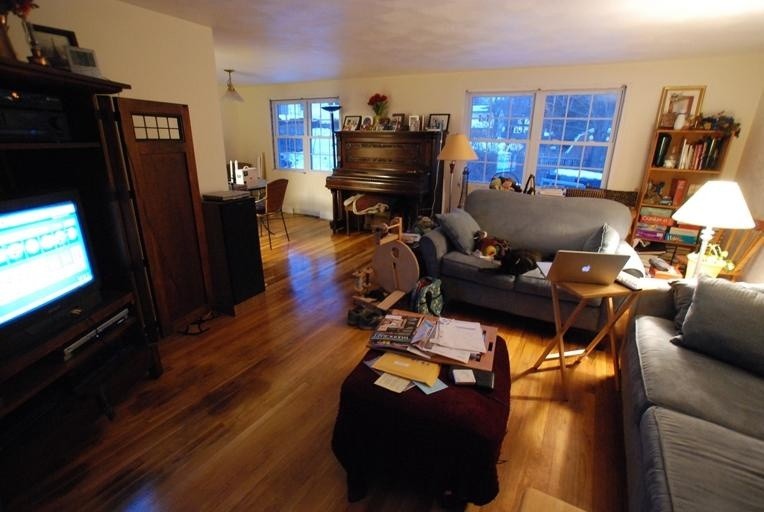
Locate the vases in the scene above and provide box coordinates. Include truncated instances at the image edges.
[685,253,726,281]
[674,113,685,130]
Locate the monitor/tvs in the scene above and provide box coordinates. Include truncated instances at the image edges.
[0,188,103,362]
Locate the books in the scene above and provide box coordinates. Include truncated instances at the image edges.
[635,127,726,278]
[358,310,498,396]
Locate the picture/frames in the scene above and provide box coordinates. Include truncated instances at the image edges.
[341,112,450,132]
[656,85,706,130]
[26,23,80,56]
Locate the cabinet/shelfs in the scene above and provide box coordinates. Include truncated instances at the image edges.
[630,130,734,279]
[0,56,161,444]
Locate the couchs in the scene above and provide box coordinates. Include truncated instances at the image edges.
[619,283,764,512]
[419,190,645,351]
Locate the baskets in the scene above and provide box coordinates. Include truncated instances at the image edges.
[565,188,606,198]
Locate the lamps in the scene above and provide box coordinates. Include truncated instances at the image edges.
[671,180,757,279]
[219,70,245,103]
[320,106,343,227]
[434,133,479,214]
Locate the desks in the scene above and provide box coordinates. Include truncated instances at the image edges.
[229,180,270,237]
[533,279,671,403]
[331,317,511,511]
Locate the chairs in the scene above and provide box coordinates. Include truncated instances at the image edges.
[675,219,764,283]
[226,162,251,184]
[256,178,289,252]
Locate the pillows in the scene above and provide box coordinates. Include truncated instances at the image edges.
[583,222,621,254]
[670,274,764,381]
[434,208,484,257]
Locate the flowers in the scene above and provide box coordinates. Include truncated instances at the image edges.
[0,0,39,21]
[367,93,390,116]
[693,243,735,272]
[490,177,523,192]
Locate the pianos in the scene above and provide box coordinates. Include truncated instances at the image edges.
[324,129,450,236]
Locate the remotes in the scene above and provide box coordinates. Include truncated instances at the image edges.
[616,271,644,291]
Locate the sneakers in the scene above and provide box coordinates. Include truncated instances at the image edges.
[359,309,383,330]
[348,303,371,325]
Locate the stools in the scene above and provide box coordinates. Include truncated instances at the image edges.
[648,264,684,279]
[344,204,391,237]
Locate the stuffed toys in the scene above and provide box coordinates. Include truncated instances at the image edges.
[472,230,489,252]
[479,239,513,260]
[490,170,536,196]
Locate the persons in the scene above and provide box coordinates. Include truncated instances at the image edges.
[362,117,372,128]
[391,116,401,126]
[382,118,395,130]
[348,119,359,128]
[346,118,351,128]
[410,118,418,130]
[432,116,444,129]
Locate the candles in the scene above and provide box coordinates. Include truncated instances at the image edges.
[229,159,239,182]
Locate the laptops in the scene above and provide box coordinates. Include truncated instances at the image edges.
[536,250,631,285]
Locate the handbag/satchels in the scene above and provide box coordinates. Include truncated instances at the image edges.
[410,276,444,316]
[493,248,538,275]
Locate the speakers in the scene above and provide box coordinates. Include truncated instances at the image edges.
[200,196,266,305]
